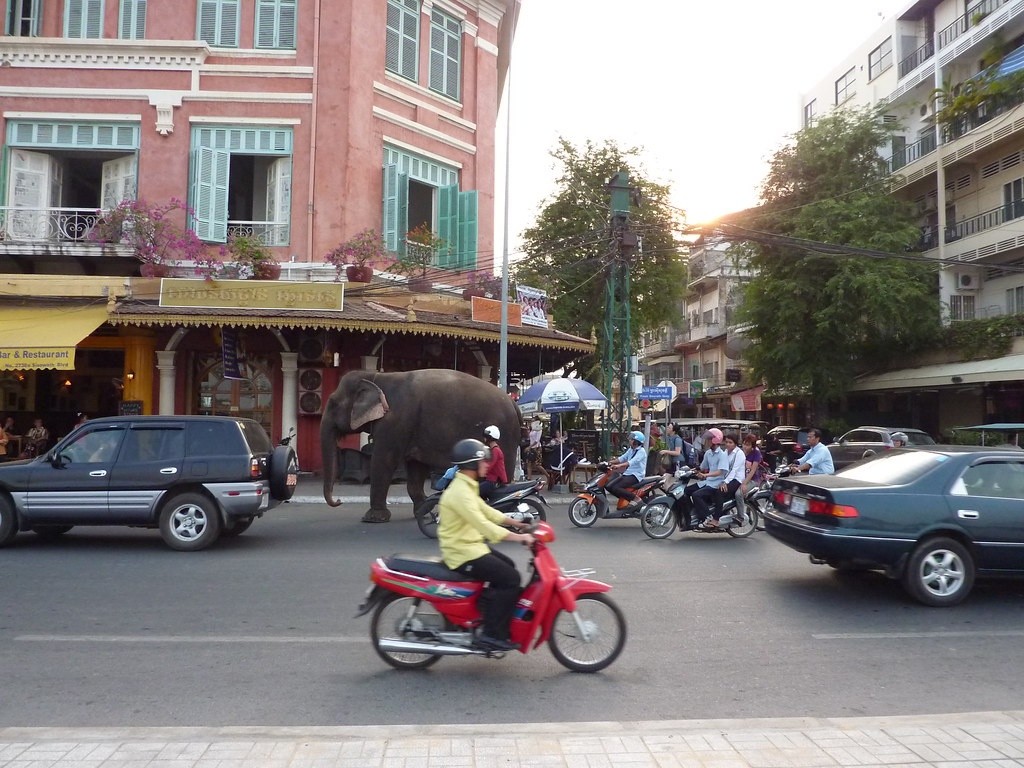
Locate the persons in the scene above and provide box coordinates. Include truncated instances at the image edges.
[478,425,508,501]
[437,439,539,652]
[0,416,48,462]
[605,431,648,511]
[889,432,909,447]
[89,435,118,466]
[520,297,546,319]
[521,415,577,491]
[788,428,835,477]
[73,414,92,448]
[658,422,788,530]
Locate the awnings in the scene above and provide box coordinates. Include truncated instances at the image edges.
[0,300,111,371]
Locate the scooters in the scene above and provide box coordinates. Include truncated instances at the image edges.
[275,427,296,447]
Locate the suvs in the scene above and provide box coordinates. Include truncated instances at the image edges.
[0,416,298,553]
[825,425,937,472]
[756,425,833,477]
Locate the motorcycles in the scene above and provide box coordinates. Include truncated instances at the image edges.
[746,456,800,530]
[351,501,628,674]
[416,466,551,541]
[639,464,759,540]
[567,455,667,527]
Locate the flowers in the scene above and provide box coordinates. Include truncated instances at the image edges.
[320,221,447,291]
[464,269,515,301]
[75,186,279,282]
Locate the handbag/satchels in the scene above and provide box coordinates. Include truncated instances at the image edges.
[662,453,672,469]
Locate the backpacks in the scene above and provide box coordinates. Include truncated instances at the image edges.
[674,436,700,469]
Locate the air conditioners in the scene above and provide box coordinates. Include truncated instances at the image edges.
[298,368,323,415]
[957,273,983,292]
[299,334,325,362]
[907,190,955,217]
[919,101,934,122]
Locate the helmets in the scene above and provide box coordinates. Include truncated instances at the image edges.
[451,439,490,470]
[890,431,908,442]
[484,425,501,439]
[629,431,645,444]
[703,428,723,445]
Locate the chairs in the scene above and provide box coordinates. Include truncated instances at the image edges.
[544,449,576,491]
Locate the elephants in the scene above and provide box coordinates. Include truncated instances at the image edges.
[319,367,530,524]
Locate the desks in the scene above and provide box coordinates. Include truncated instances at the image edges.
[7,435,26,459]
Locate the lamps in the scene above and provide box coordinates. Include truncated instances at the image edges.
[127,368,135,383]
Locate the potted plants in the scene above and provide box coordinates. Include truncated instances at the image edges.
[927,63,1024,138]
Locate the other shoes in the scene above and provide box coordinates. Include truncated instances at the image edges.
[698,514,713,528]
[706,519,721,527]
[628,499,643,508]
[479,633,520,650]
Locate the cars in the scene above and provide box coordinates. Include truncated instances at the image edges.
[763,446,1023,610]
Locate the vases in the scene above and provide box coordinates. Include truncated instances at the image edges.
[463,289,485,301]
[141,263,169,279]
[346,265,373,282]
[253,263,282,280]
[408,278,433,294]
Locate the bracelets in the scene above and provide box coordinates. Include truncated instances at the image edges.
[99,446,103,451]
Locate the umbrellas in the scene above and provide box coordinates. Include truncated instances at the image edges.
[517,376,610,485]
